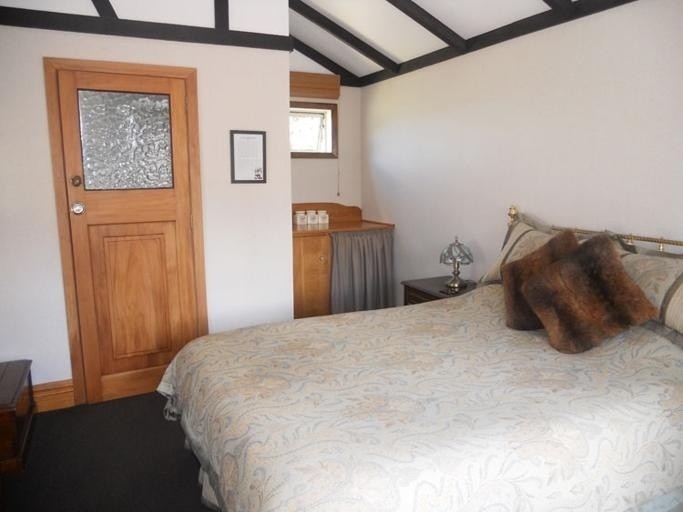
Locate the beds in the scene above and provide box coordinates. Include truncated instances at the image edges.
[157,206,683,511]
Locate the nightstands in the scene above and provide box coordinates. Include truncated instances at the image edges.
[401,276,477,307]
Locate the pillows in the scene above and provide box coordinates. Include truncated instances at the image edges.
[477,219,683,341]
[522,236,657,352]
[504,236,578,329]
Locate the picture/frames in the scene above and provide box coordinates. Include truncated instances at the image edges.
[230,131,265,183]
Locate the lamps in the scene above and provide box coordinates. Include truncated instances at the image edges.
[439,236,472,287]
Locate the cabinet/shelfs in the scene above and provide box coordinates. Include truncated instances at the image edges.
[293,202,394,317]
[0,360,37,477]
